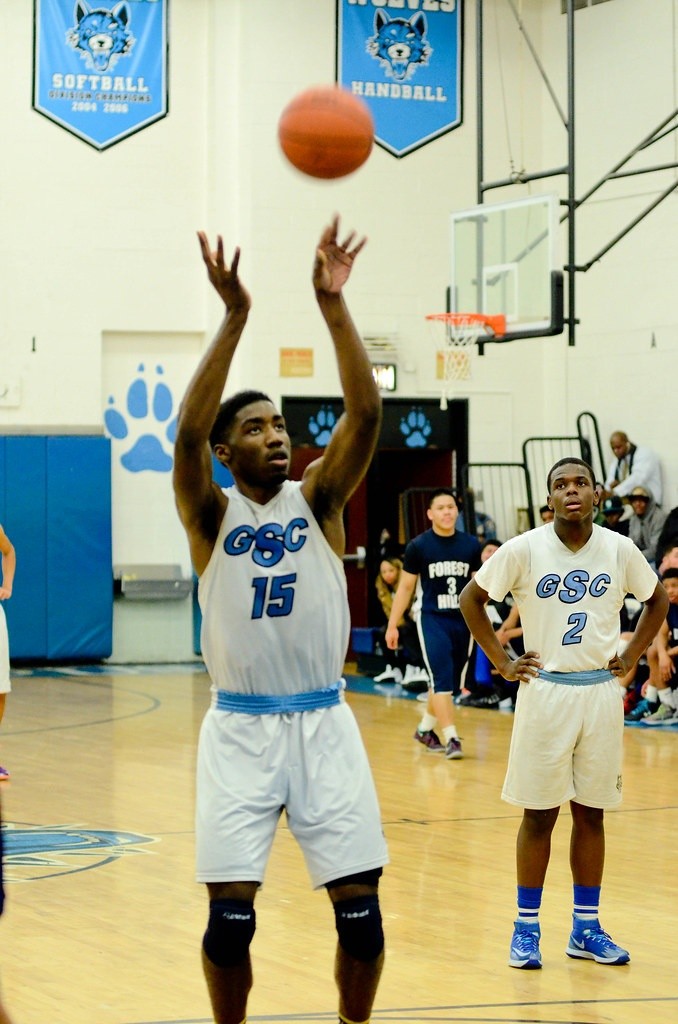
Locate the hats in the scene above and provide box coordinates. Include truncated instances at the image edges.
[600,496,625,515]
[628,487,651,503]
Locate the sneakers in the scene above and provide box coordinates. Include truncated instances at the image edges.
[0,767,10,780]
[400,664,430,702]
[624,695,661,724]
[509,917,544,970]
[460,688,512,710]
[412,728,446,753]
[373,664,403,683]
[640,702,678,726]
[567,914,631,964]
[624,681,643,716]
[445,736,464,759]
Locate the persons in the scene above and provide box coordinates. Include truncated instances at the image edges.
[374,431,678,727]
[458,458,669,971]
[173,214,384,1023]
[384,487,483,760]
[0,525,15,780]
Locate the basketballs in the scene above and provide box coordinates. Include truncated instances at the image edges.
[278,87,375,178]
